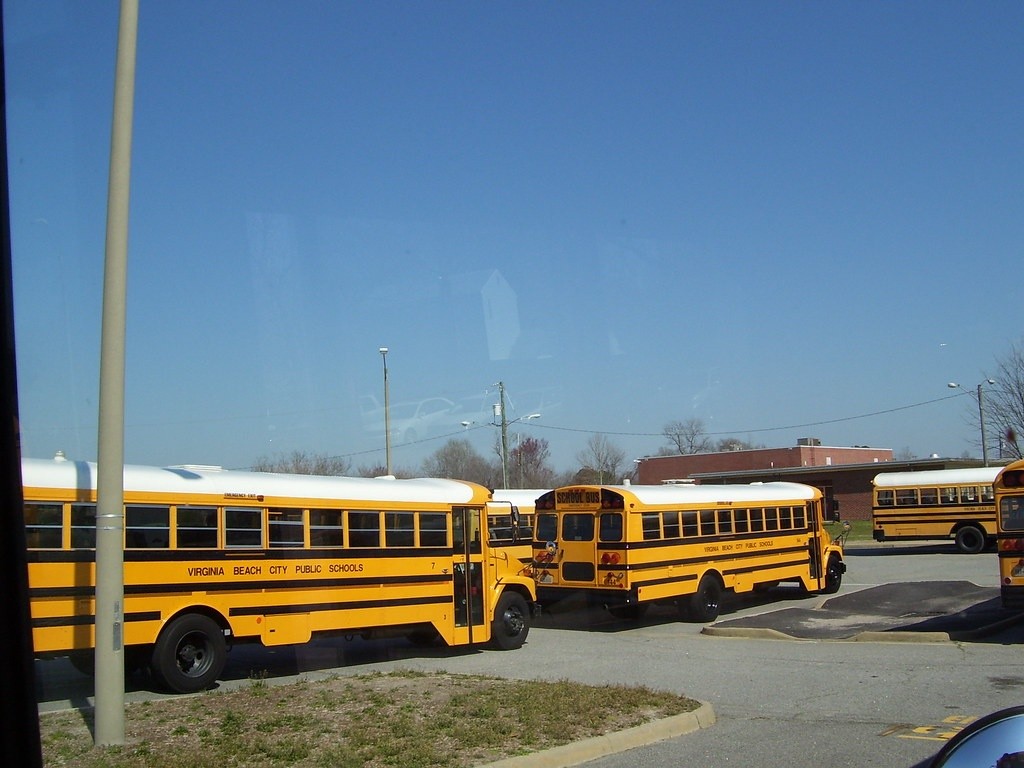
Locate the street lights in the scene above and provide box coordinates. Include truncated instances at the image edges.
[379,346,392,478]
[947,378,996,466]
[462,414,543,488]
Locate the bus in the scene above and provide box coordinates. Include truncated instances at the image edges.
[871,466,1016,554]
[470,484,584,596]
[21,456,540,695]
[531,477,852,626]
[991,457,1024,611]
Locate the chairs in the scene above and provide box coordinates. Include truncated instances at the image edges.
[880,495,988,504]
